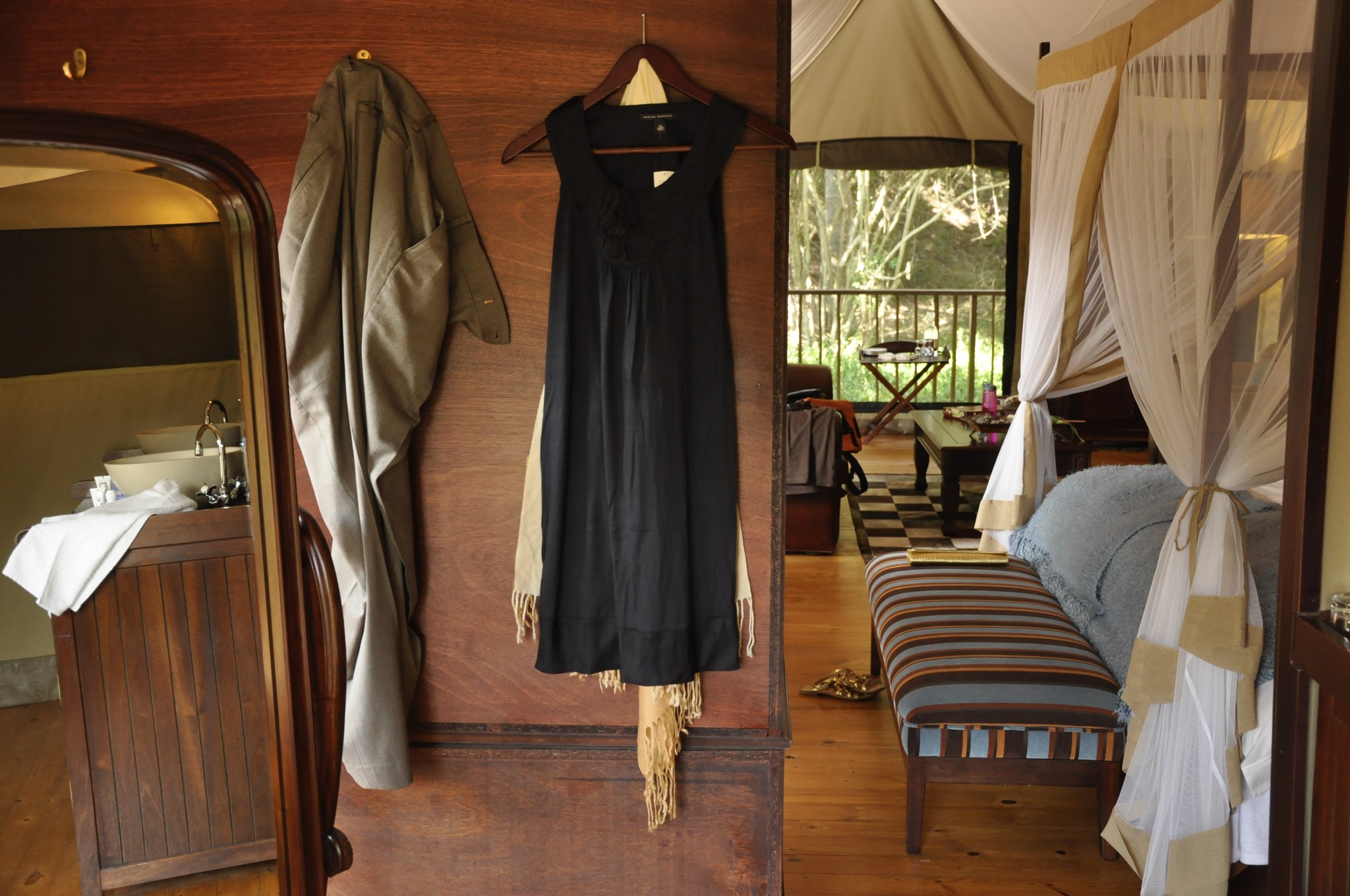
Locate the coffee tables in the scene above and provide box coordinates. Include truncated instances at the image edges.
[907,404,1099,538]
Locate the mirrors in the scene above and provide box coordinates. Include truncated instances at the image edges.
[0,99,358,896]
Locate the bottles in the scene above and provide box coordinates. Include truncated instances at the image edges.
[982,381,997,417]
[923,321,939,356]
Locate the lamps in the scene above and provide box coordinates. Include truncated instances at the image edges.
[923,324,939,358]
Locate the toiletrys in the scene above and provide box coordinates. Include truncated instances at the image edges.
[89,475,125,507]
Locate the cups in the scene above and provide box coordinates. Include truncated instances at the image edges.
[1329,592,1350,637]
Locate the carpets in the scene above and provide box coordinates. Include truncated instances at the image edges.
[844,472,991,566]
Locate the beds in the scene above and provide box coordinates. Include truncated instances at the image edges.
[1003,0,1350,896]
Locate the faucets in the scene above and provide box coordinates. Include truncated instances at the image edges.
[204,397,230,426]
[193,423,231,506]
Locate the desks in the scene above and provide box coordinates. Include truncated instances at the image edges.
[856,350,950,447]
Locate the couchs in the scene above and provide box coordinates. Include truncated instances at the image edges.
[781,360,844,559]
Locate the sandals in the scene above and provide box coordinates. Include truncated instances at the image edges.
[801,667,885,700]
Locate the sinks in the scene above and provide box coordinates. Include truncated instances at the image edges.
[102,444,246,498]
[133,421,241,455]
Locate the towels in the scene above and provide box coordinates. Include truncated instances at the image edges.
[1,477,199,617]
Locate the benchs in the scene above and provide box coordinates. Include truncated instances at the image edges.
[861,547,1128,863]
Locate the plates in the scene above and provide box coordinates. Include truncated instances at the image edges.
[863,348,887,356]
[960,414,1015,433]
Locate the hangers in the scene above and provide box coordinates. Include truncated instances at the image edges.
[493,13,798,167]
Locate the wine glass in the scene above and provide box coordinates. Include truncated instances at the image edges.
[914,340,934,356]
